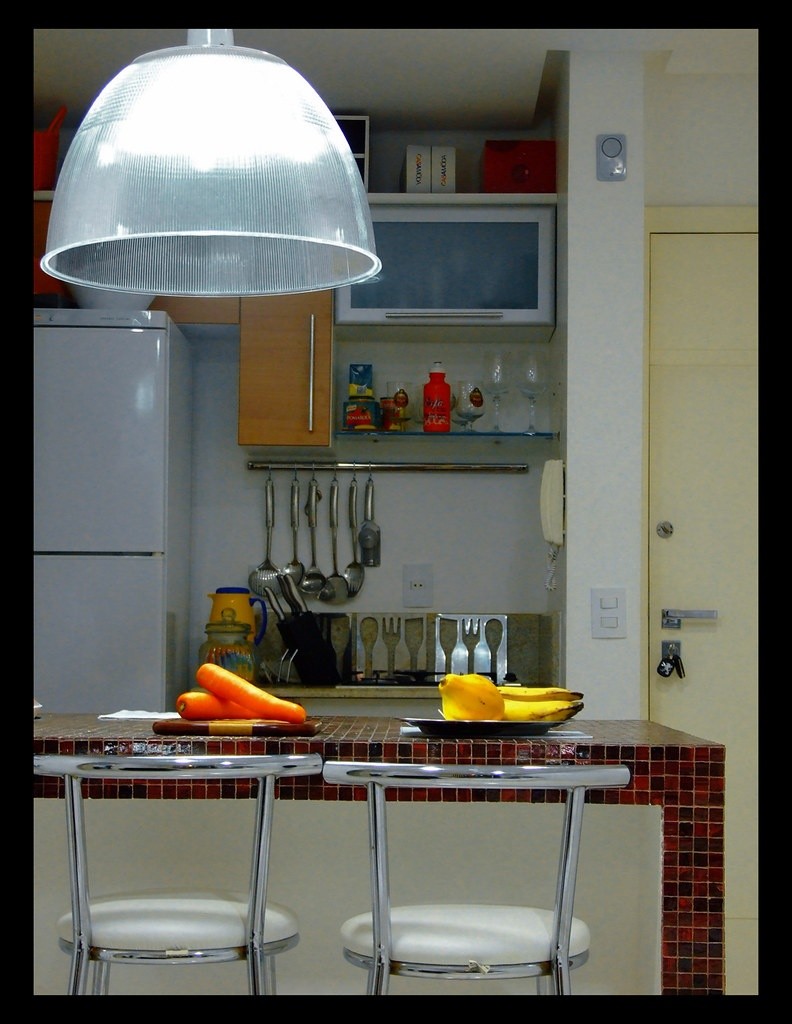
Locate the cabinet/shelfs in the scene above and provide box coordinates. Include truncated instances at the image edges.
[143,201,557,448]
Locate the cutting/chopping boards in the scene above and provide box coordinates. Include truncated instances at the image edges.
[153,718,322,737]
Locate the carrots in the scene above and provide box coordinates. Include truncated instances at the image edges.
[196,663,307,723]
[176,692,270,720]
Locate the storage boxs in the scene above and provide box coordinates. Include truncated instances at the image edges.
[333,112,370,192]
[398,145,431,192]
[432,144,457,193]
[479,137,557,194]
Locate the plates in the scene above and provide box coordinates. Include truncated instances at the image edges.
[395,718,572,739]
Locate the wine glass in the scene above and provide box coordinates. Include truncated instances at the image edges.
[481,350,548,433]
[387,381,415,431]
[412,386,424,432]
[452,380,486,433]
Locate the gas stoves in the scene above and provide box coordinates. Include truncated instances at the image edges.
[336,671,521,688]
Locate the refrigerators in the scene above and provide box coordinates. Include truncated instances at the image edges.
[33,309,192,714]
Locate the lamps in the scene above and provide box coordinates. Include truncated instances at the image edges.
[39,29,380,297]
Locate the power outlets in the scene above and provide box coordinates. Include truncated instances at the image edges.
[401,562,434,606]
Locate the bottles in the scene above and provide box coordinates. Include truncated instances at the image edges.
[198,607,259,685]
[423,362,451,432]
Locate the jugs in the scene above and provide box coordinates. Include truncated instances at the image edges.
[207,587,267,647]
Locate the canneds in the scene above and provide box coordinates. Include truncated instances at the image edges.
[343,395,405,431]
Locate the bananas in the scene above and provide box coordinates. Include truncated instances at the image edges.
[495,686,586,722]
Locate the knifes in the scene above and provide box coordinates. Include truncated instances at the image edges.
[264,574,308,618]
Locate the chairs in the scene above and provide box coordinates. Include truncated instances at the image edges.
[322,761,629,994]
[34,750,323,995]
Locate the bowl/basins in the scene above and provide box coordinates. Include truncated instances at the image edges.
[69,284,154,309]
